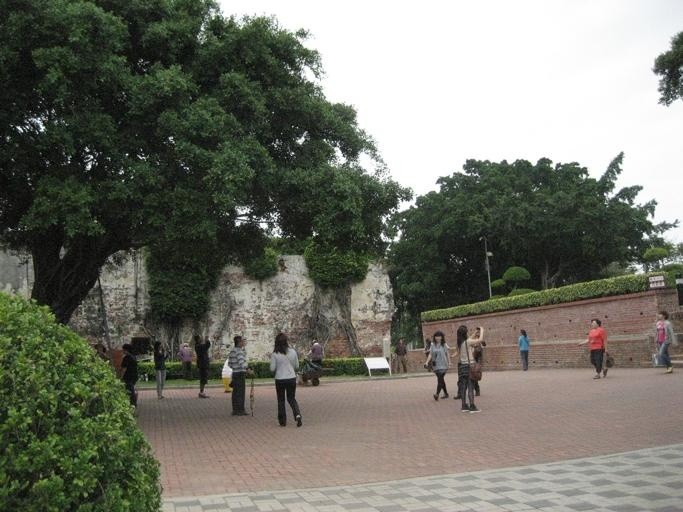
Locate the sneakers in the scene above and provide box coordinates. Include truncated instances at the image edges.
[593,376,600,379]
[667,366,673,373]
[461,404,469,412]
[664,368,669,374]
[603,369,607,377]
[470,405,481,413]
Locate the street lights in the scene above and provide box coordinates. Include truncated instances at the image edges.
[481,237,495,301]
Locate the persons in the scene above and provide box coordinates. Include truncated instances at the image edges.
[154,340,171,401]
[228,336,255,416]
[653,310,679,374]
[94,343,112,366]
[424,331,452,400]
[455,325,486,413]
[305,339,325,378]
[423,338,434,372]
[178,342,195,381]
[451,331,482,400]
[517,329,530,371]
[270,332,303,427]
[118,343,139,410]
[221,356,233,393]
[576,318,608,380]
[193,333,211,399]
[394,338,409,373]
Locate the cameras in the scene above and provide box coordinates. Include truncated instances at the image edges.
[476,328,480,330]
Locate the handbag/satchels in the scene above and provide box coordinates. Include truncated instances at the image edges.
[469,363,482,381]
[606,352,614,368]
[653,347,664,366]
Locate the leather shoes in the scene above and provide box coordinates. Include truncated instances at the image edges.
[199,393,251,416]
[296,415,302,427]
[433,391,480,400]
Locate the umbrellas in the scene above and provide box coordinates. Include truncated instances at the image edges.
[248,372,257,417]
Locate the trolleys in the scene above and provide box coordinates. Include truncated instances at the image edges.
[297,363,324,385]
